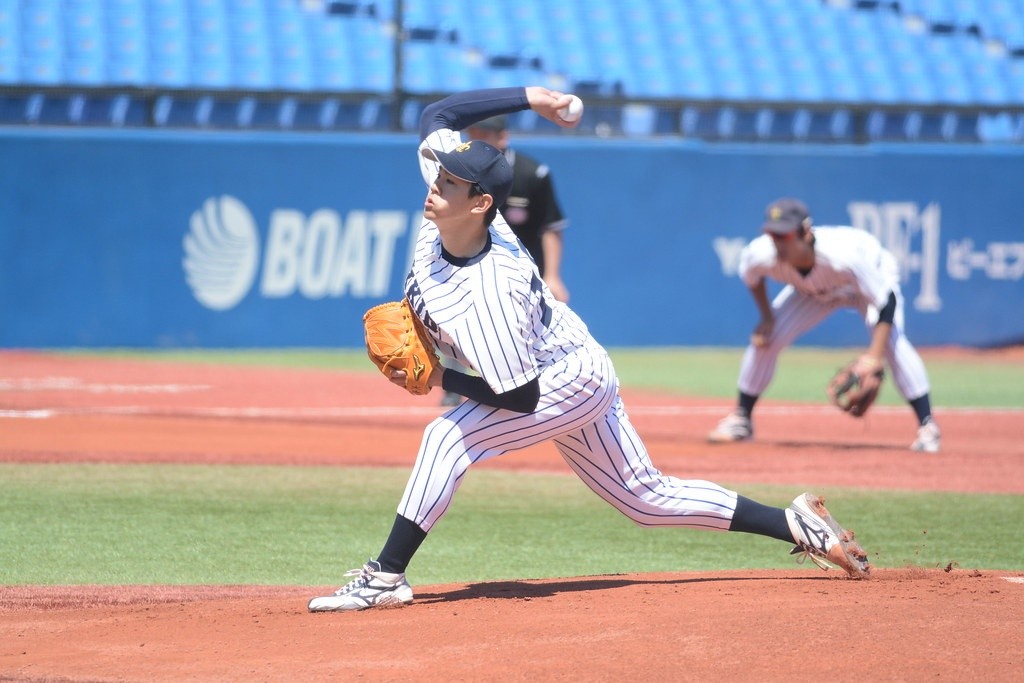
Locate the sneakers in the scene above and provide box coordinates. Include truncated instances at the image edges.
[708,415,752,443]
[910,423,940,453]
[785,492,870,578]
[308,557,413,611]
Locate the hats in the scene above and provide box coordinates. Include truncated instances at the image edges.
[761,198,808,233]
[422,140,514,208]
[478,115,508,130]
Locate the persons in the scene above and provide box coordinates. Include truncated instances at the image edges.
[707,198,941,451]
[441,116,570,407]
[309,87,870,613]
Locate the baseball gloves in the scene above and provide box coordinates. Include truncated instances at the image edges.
[362,298,442,397]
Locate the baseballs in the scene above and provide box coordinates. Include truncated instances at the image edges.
[556,95,584,122]
